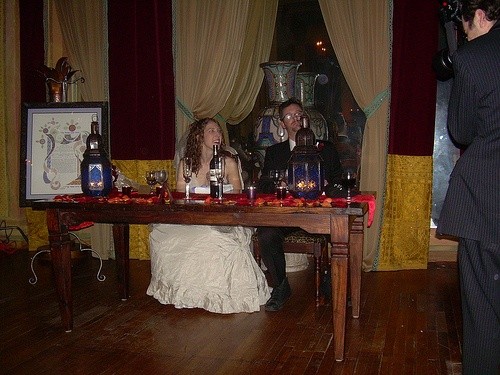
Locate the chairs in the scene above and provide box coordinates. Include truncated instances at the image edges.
[237,143,328,310]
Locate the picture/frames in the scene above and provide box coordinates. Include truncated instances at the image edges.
[17,99,109,206]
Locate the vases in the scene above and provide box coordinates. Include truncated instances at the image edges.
[48,79,69,102]
[255,59,302,146]
[295,71,328,142]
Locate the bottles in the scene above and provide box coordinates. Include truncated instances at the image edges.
[81,121,113,196]
[210,145,224,198]
[252,60,304,171]
[287,116,321,199]
[295,71,328,149]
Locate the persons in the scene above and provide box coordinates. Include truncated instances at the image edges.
[147,117,272,314]
[437,0,500,375]
[257,98,344,312]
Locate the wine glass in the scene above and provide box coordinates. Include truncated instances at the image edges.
[340,173,356,203]
[146,168,157,196]
[183,157,193,200]
[155,169,168,196]
[214,155,227,201]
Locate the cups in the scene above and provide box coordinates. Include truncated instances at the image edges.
[277,186,286,198]
[245,182,257,199]
[274,168,288,195]
[122,186,131,195]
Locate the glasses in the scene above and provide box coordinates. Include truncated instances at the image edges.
[280,110,304,120]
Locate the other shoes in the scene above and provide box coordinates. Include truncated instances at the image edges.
[321,265,352,306]
[265,275,291,312]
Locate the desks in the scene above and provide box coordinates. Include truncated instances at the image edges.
[36,187,377,362]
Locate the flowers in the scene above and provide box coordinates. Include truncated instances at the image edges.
[38,57,85,85]
